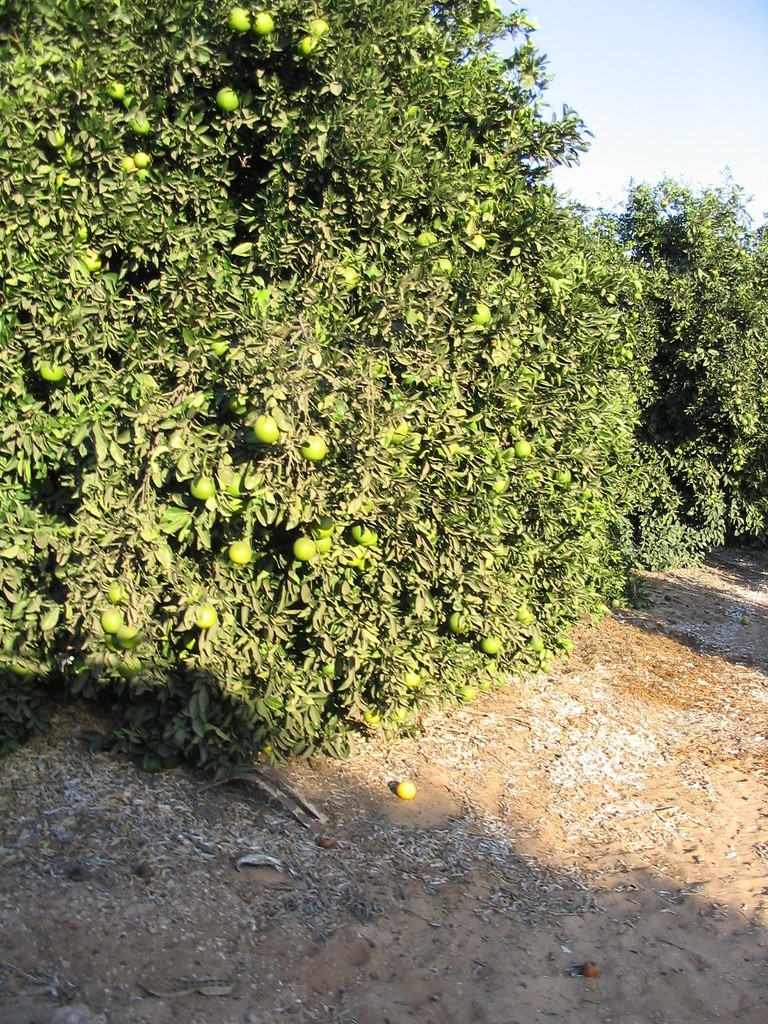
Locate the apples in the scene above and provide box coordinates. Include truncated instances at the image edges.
[36,11,754,800]
